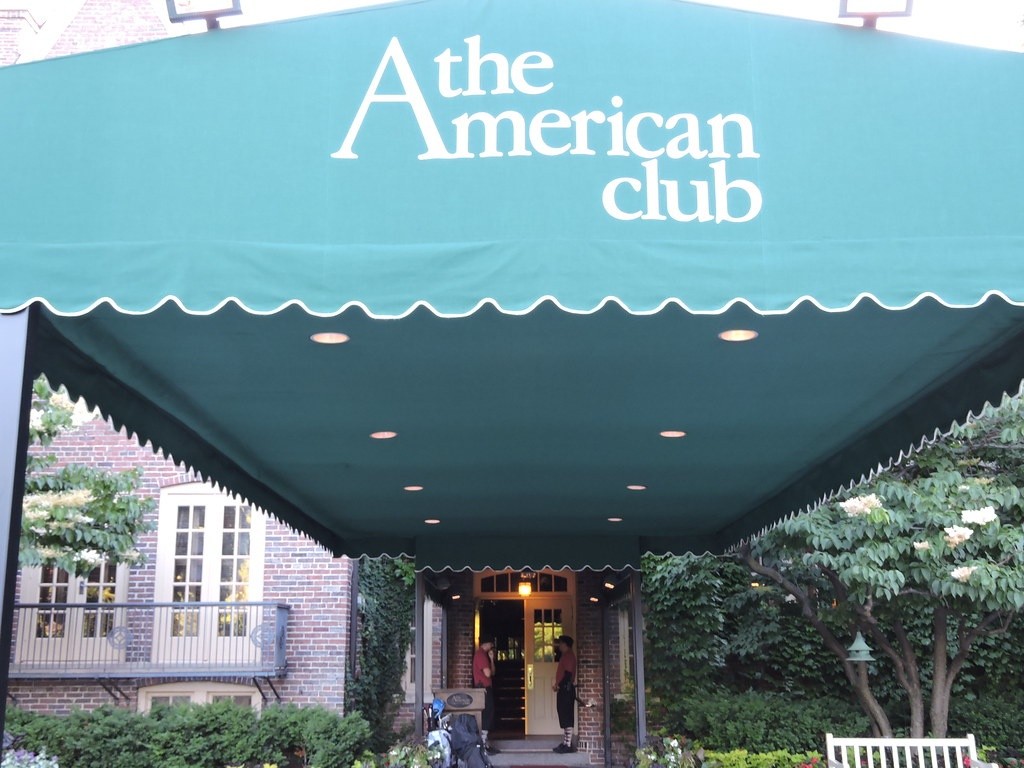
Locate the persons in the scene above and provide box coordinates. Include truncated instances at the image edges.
[551,635,576,753]
[472,634,502,755]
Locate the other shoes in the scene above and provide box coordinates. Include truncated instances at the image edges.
[483,743,500,756]
[553,743,577,753]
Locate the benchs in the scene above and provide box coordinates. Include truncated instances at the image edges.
[825,730,1000,768]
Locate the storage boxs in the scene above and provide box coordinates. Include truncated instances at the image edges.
[441,711,481,737]
[431,688,486,711]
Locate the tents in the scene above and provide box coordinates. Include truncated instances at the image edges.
[0,0,1024,768]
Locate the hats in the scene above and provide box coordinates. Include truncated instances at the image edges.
[479,634,495,643]
[559,636,573,645]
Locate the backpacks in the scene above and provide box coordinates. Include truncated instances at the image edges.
[426,730,457,768]
